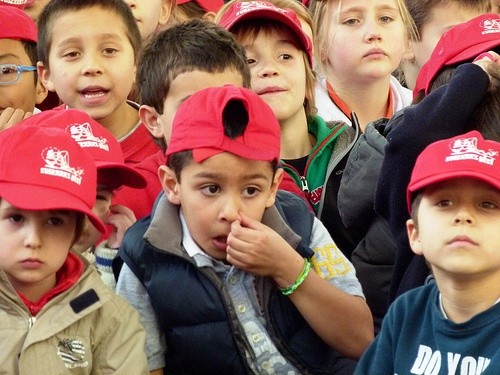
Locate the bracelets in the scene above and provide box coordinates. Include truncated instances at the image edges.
[279,257,311,296]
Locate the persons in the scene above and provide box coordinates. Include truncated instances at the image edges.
[116,84,374,375]
[0,0,500,375]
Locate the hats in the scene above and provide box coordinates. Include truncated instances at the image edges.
[217,0,313,72]
[0,123,108,234]
[406,130,500,216]
[413,60,430,102]
[175,0,224,15]
[0,5,39,42]
[164,84,280,163]
[424,12,500,96]
[19,108,147,189]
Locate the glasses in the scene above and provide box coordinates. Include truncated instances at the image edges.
[0,63,37,86]
